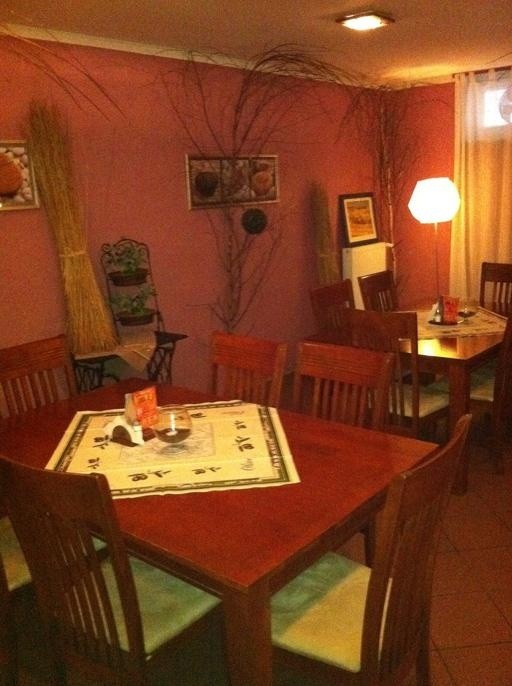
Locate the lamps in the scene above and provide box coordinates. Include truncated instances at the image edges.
[334,11,397,31]
[406,177,460,298]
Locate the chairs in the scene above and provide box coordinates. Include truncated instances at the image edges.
[1,334,81,418]
[1,514,108,686]
[208,331,289,408]
[0,455,222,686]
[291,339,395,566]
[269,413,473,686]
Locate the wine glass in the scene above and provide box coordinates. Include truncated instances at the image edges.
[154,403,193,455]
[456,295,481,325]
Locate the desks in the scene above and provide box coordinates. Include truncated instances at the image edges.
[73,331,189,392]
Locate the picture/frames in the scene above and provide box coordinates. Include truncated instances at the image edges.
[339,192,381,248]
[1,139,41,211]
[185,153,282,210]
